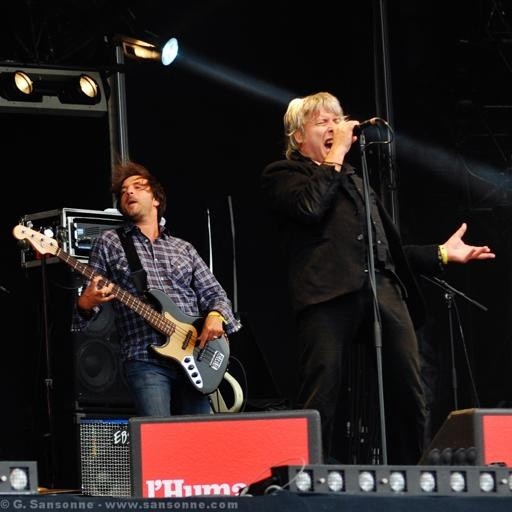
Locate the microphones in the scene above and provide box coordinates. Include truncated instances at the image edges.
[350,118,377,135]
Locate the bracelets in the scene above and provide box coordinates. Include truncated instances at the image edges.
[438,245,448,264]
[208,311,224,322]
[323,161,343,167]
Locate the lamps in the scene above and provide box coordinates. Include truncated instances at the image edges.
[103,27,180,68]
[68,74,101,103]
[2,69,36,100]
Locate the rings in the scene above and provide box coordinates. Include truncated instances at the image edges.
[213,336,218,340]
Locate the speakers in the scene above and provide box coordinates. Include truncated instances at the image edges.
[411,408,512,468]
[78,408,325,500]
[55,280,139,411]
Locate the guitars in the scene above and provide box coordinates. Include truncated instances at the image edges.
[12,216,231,395]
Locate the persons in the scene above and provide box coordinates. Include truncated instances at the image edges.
[70,162,243,418]
[263,91,497,466]
